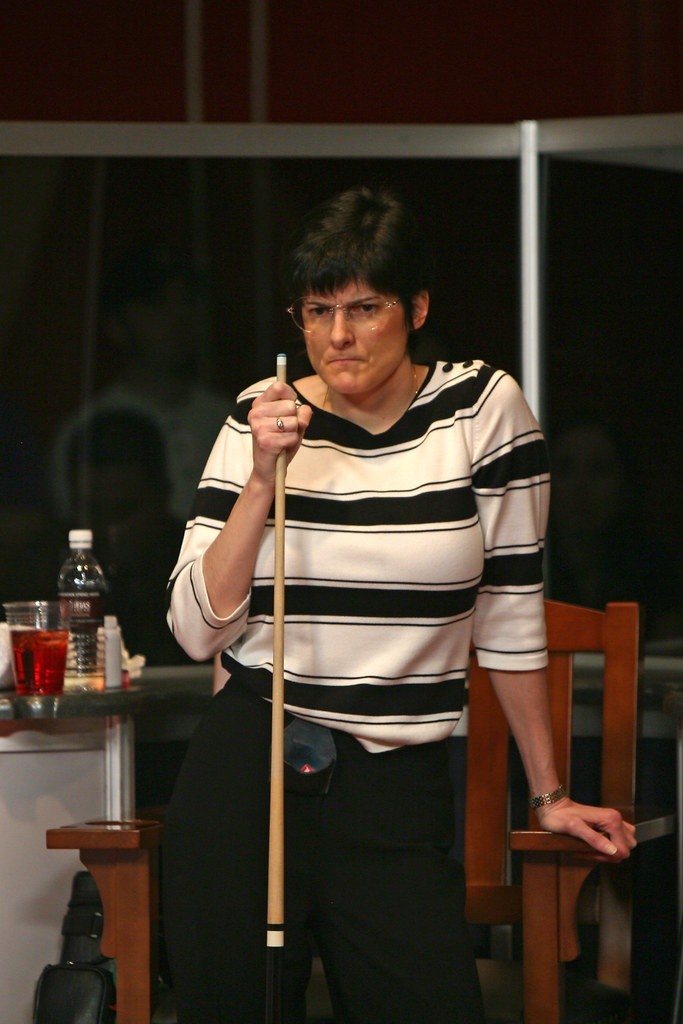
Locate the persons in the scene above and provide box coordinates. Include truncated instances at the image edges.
[163,188,637,1024]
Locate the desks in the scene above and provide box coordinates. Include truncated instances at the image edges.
[0,650,215,1024]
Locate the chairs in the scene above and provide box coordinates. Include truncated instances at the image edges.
[46,600,683,1024]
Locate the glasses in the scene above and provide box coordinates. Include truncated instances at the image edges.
[287,295,401,335]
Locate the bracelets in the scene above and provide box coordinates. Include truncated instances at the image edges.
[528,784,565,808]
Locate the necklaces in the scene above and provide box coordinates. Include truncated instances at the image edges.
[322,365,417,410]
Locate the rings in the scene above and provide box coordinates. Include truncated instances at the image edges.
[276,417,283,432]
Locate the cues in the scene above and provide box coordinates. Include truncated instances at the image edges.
[261,350,288,1023]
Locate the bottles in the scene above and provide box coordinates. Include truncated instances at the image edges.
[105,616,122,688]
[60,529,104,690]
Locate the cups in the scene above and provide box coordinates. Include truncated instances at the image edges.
[2,601,75,694]
